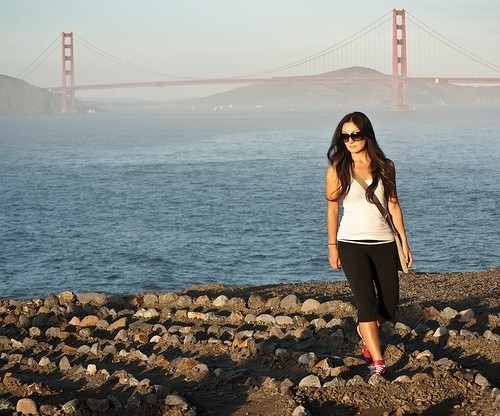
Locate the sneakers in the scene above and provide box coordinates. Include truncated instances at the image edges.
[369,365,385,386]
[357,325,375,358]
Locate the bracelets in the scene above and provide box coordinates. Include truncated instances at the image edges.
[328,244,337,245]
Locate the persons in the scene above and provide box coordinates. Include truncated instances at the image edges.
[325,111,413,381]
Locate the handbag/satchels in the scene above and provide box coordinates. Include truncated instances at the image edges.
[393,234,408,274]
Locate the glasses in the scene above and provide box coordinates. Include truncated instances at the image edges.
[341,132,364,143]
